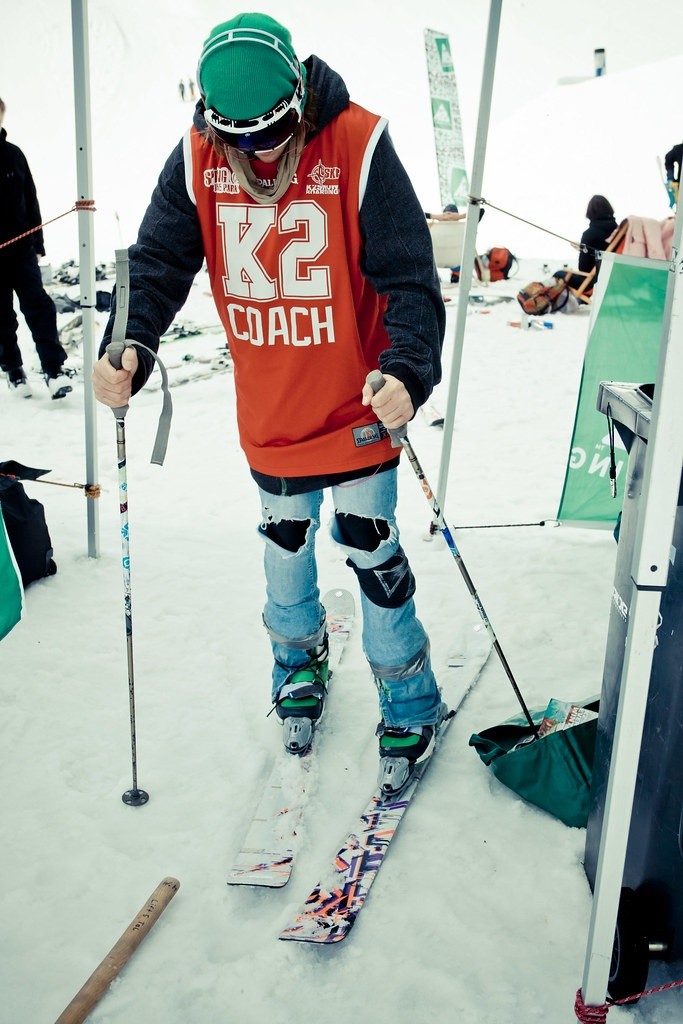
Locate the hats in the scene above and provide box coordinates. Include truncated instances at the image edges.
[197,13,307,121]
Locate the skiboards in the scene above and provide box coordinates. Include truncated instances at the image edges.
[224,586,493,944]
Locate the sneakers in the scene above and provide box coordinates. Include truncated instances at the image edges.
[5,368,33,398]
[43,371,74,401]
[375,722,439,762]
[274,656,323,720]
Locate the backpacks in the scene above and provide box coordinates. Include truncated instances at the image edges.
[517,274,570,315]
[474,247,518,283]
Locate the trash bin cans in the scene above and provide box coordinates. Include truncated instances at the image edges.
[583,381,683,1002]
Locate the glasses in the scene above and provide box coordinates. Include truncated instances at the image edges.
[205,106,301,152]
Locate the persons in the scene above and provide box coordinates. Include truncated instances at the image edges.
[423,204,484,284]
[93,13,448,797]
[553,195,618,291]
[0,98,74,400]
